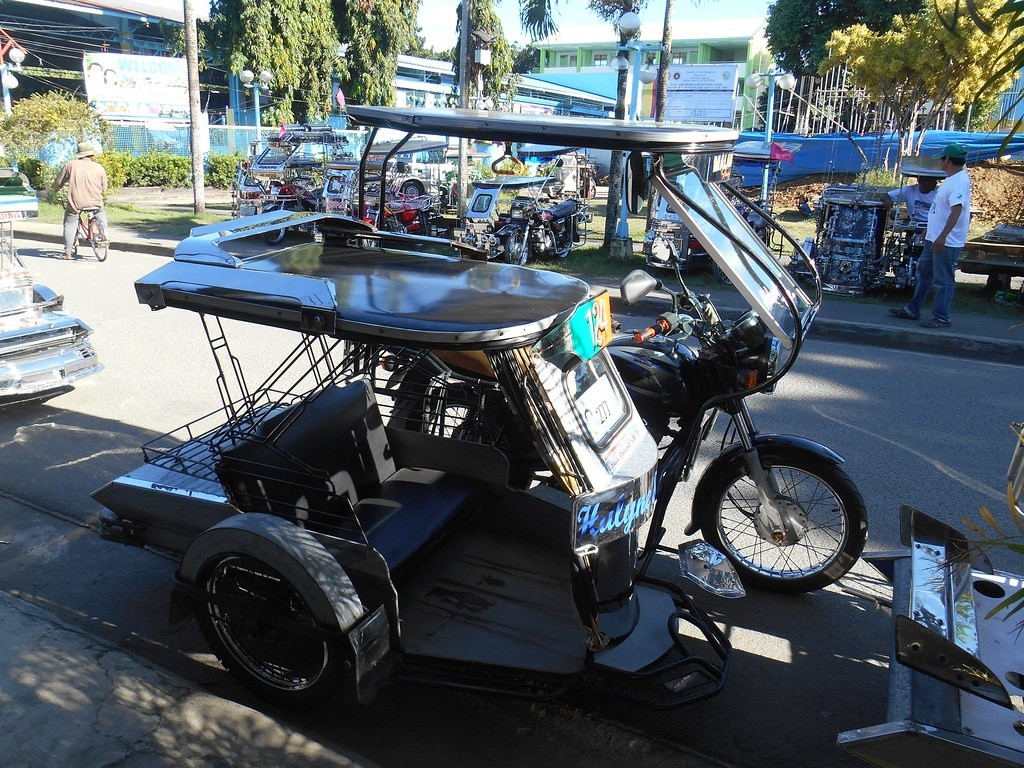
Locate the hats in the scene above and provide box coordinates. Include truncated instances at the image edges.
[933,143,968,158]
[76,142,97,158]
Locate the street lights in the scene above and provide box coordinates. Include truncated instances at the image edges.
[0,47,26,116]
[606,10,668,261]
[745,70,797,207]
[239,68,275,155]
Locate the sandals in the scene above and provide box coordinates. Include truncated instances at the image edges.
[920,317,951,329]
[888,307,920,320]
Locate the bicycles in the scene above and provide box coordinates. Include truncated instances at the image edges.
[56,195,111,262]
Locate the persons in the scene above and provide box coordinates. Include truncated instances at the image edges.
[888,178,941,284]
[51,143,107,260]
[889,144,969,328]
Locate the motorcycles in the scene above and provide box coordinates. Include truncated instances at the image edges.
[791,176,927,297]
[101,97,872,726]
[833,418,1024,768]
[0,166,42,219]
[0,222,104,420]
[640,180,788,287]
[230,126,455,254]
[455,155,595,267]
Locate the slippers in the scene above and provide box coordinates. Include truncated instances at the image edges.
[56,252,73,260]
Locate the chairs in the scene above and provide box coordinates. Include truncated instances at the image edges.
[215,374,489,586]
